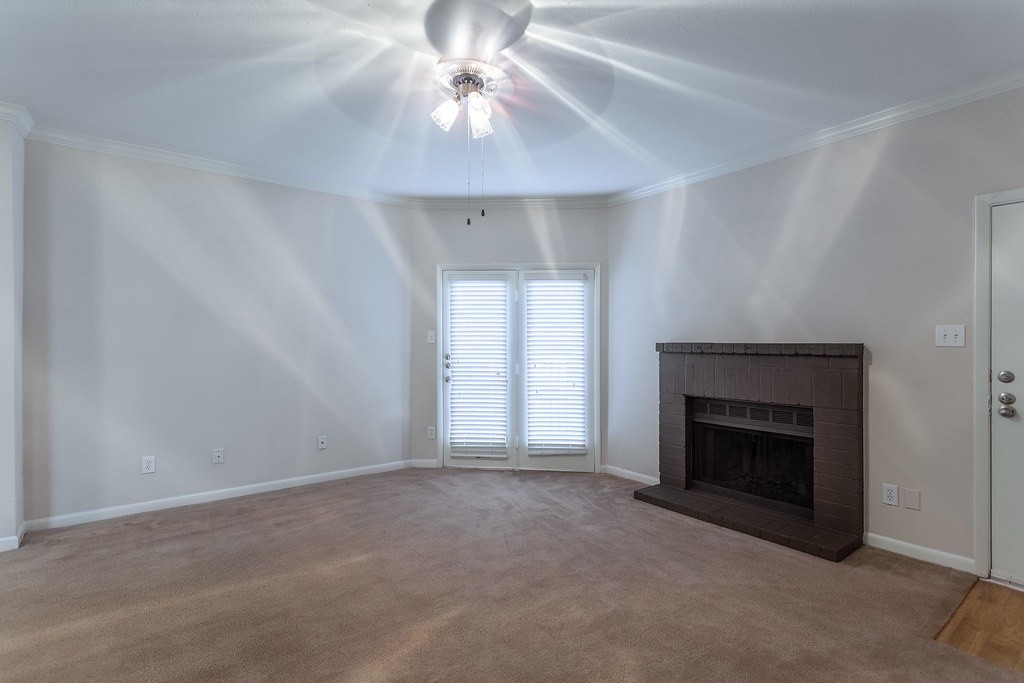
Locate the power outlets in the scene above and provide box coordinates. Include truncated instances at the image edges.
[882,484,899,506]
[214,448,224,463]
[142,456,156,474]
[428,427,435,439]
[318,436,327,450]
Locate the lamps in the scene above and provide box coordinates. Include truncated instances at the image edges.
[433,0,500,139]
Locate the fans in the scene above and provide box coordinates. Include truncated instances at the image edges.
[315,0,615,158]
